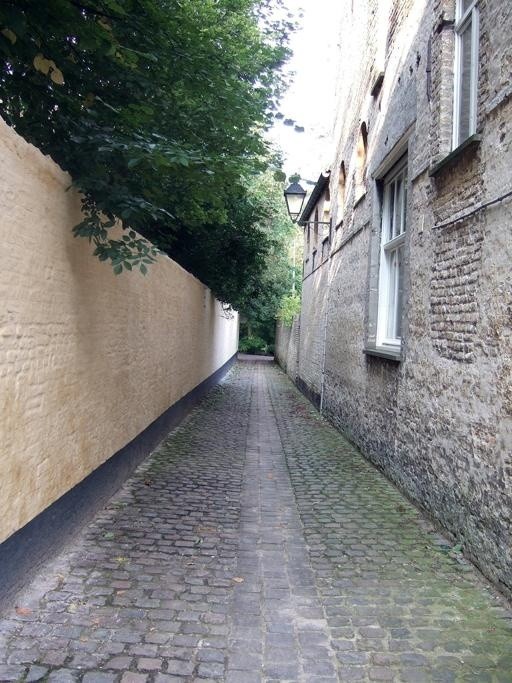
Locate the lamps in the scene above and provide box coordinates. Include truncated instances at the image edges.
[285,174,334,227]
